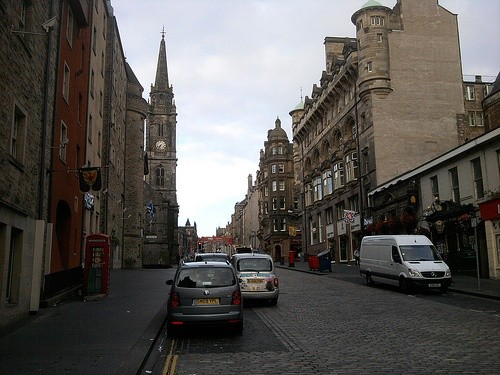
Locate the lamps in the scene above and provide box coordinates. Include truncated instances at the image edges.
[121,214,132,219]
[99,188,110,196]
[115,207,128,214]
[11,15,62,37]
[112,199,122,207]
[47,137,71,149]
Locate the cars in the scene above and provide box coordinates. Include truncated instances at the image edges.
[166,260,245,337]
[230,253,280,306]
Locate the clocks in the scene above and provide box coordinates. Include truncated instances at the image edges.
[155,140,166,151]
[434,204,443,211]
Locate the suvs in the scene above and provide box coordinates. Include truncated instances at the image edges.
[194,251,232,264]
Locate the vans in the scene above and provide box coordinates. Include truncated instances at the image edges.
[360,235,453,293]
